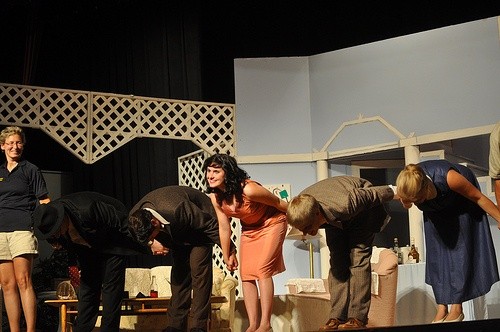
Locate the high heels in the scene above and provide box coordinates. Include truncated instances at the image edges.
[431,311,449,323]
[442,311,465,324]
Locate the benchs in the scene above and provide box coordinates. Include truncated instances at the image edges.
[44,297,227,332]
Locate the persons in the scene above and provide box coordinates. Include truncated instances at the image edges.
[0,127,51,332]
[35,193,169,331]
[396,160,500,324]
[286,175,413,329]
[129,185,238,332]
[201,154,289,331]
[489,124,500,230]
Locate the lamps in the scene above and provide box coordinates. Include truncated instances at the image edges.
[288,217,321,276]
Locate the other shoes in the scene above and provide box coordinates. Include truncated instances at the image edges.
[161,326,180,332]
[188,328,203,332]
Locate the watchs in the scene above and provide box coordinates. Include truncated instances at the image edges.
[147,239,154,247]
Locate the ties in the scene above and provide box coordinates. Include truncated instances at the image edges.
[60,231,80,289]
[159,225,170,238]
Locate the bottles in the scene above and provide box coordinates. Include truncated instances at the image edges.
[149,276,158,298]
[394,238,402,264]
[407,239,419,263]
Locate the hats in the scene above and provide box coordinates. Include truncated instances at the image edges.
[31,200,65,242]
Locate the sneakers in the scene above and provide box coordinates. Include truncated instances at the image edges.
[338,318,368,332]
[318,319,346,332]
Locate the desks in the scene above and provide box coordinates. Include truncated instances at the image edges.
[396,262,488,326]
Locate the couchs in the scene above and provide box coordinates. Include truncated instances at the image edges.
[234,246,400,332]
[149,266,239,332]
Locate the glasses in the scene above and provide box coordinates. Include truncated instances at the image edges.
[5,141,25,149]
